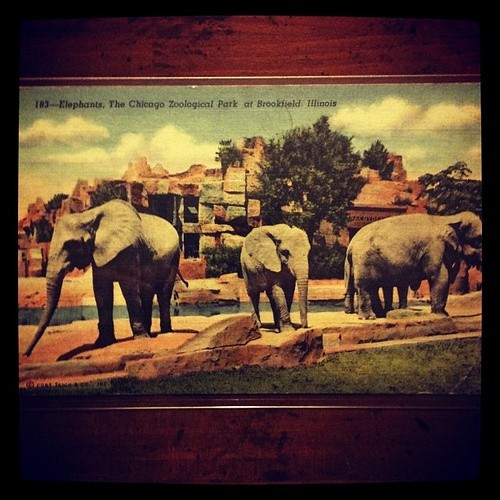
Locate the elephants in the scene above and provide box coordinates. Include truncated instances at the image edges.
[23,200,188,358]
[241,224,311,334]
[344,212,482,320]
